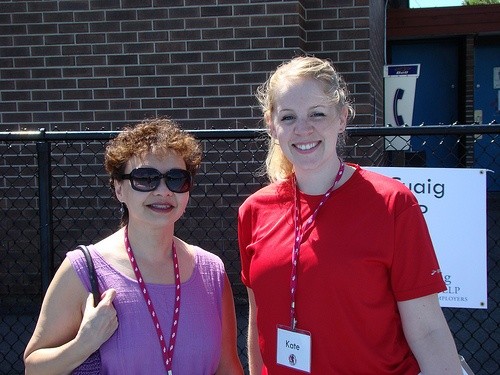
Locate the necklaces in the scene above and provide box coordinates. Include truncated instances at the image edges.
[124,224,181,375]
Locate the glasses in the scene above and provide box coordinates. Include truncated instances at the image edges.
[123,167,193,193]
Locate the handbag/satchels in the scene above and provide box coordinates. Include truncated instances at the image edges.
[71,245,101,375]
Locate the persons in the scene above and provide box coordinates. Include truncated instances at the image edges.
[23,119,243,375]
[238,57,463,375]
